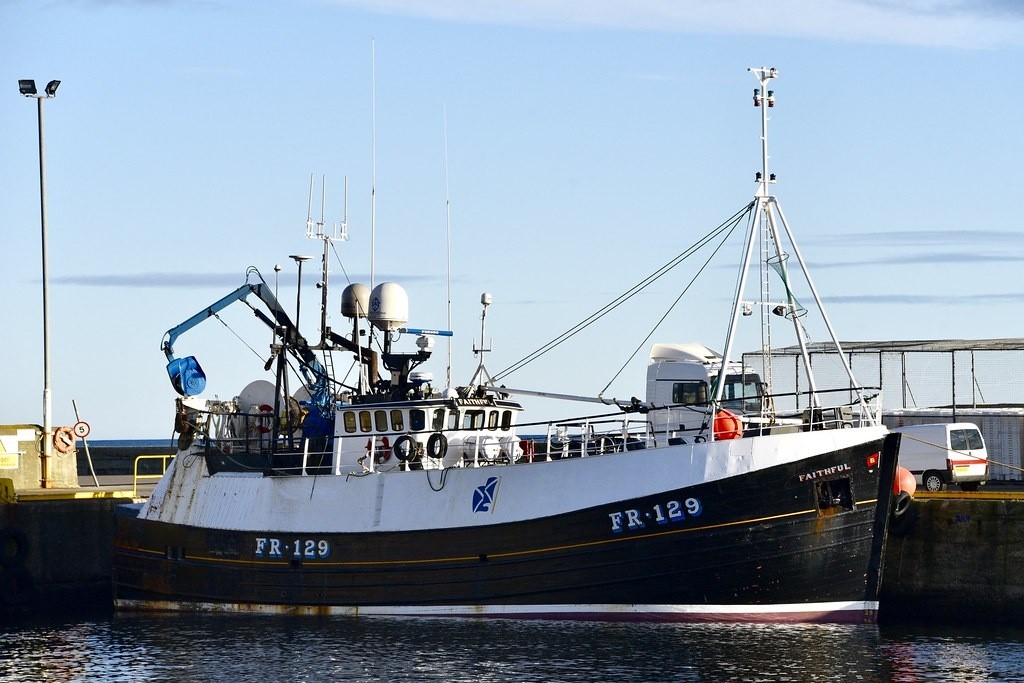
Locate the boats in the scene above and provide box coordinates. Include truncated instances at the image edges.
[105,65,907,631]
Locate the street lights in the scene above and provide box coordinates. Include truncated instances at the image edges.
[18,79,63,489]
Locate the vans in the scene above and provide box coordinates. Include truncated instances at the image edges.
[888,422,989,491]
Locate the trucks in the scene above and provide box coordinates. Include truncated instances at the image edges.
[643,344,769,449]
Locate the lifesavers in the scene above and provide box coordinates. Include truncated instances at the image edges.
[890,490,911,517]
[426,433,449,458]
[394,435,418,461]
[54,426,76,452]
[254,404,274,433]
